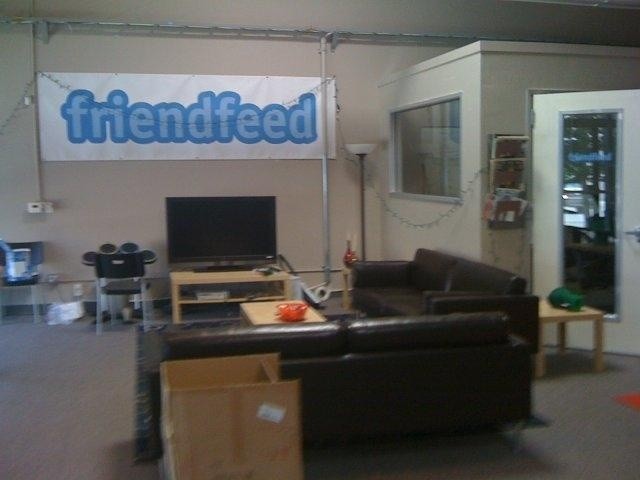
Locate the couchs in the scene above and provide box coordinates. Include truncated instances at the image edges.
[136,311,533,457]
[353,247,541,359]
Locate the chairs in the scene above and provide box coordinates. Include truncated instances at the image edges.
[92,252,157,335]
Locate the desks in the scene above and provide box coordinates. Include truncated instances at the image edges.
[238,300,326,327]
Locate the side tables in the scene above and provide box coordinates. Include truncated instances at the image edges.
[536,299,606,377]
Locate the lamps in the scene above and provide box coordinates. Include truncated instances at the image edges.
[344,142,379,260]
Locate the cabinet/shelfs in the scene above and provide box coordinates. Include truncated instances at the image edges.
[342,270,355,309]
[170,269,292,322]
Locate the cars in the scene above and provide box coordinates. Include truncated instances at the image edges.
[563,188,597,231]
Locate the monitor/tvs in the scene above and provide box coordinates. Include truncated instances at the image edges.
[166,197,277,274]
